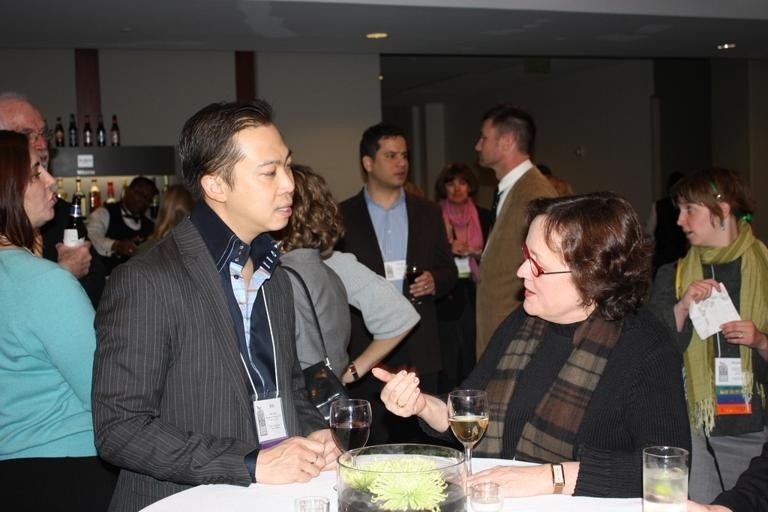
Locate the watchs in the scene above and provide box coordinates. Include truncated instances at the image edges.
[550,462,566,495]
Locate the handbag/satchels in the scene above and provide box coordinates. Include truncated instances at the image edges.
[708,385,763,436]
[303,361,350,421]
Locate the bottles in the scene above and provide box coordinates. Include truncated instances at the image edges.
[82,114,93,147]
[150,177,161,219]
[105,181,117,204]
[119,178,128,201]
[54,116,65,147]
[55,177,68,202]
[72,177,86,218]
[110,114,120,147]
[95,115,107,147]
[62,193,85,248]
[88,176,102,215]
[68,113,79,147]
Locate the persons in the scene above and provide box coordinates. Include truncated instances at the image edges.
[0,128,113,512]
[372,192,691,499]
[86,175,157,307]
[680,438,767,512]
[152,185,190,239]
[91,99,342,512]
[651,165,768,504]
[0,92,109,312]
[283,104,575,451]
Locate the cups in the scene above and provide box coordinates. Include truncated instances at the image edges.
[641,444,691,511]
[294,495,331,512]
[470,482,502,511]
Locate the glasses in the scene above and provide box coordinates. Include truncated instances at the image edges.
[25,127,55,143]
[521,239,571,277]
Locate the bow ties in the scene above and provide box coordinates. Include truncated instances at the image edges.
[123,213,140,224]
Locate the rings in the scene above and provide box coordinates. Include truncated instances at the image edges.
[740,332,744,337]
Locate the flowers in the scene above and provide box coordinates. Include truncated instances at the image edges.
[342,453,448,512]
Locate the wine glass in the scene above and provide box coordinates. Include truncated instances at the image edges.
[329,397,373,491]
[406,263,425,306]
[446,389,490,476]
